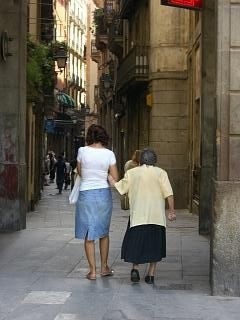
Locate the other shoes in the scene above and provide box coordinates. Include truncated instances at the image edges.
[101,268,113,276]
[145,276,154,284]
[87,272,96,280]
[131,269,140,282]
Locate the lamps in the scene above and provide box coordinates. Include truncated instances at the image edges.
[85,104,90,114]
[52,41,67,67]
[101,74,113,88]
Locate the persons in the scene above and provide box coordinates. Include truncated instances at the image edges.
[74,124,119,280]
[46,150,78,194]
[124,150,141,173]
[107,148,177,283]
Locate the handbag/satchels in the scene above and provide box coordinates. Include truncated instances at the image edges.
[68,167,81,203]
